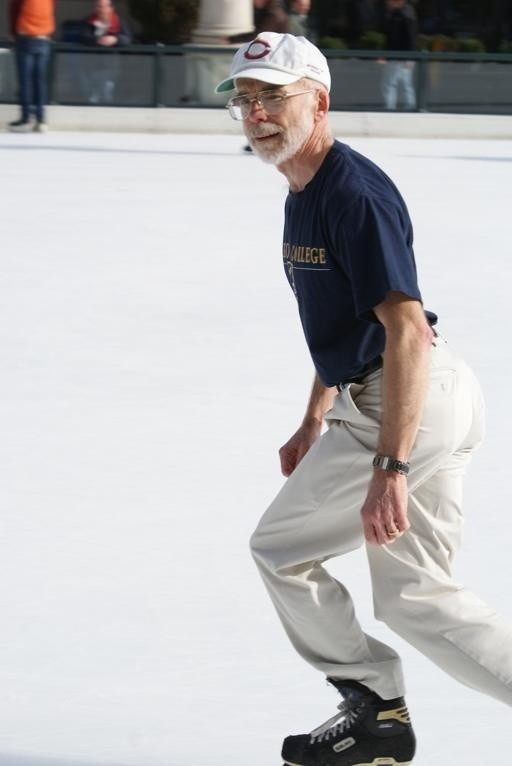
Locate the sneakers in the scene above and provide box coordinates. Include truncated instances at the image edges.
[8,119,50,133]
[281,674,414,764]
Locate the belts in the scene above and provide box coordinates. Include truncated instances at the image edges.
[340,355,383,386]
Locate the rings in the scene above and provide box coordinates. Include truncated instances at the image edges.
[386,529,399,538]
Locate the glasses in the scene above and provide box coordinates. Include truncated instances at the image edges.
[225,90,314,120]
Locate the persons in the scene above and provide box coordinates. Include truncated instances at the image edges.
[212,31,511,766]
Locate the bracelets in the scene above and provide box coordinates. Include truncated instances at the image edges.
[370,453,410,478]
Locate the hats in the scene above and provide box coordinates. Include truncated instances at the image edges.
[215,31,331,94]
